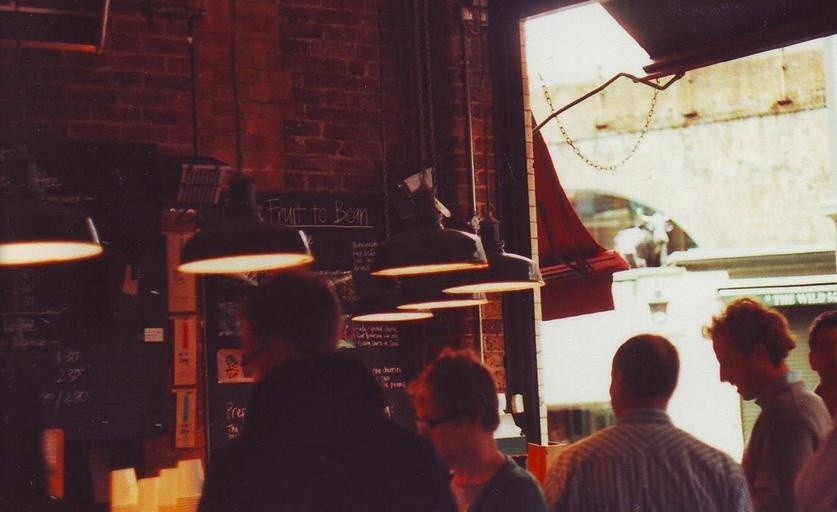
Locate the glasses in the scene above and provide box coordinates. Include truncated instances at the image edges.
[413,410,464,433]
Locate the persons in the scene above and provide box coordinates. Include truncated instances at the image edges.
[709,298,833,512]
[542,334,755,512]
[403,345,546,512]
[807,310,837,416]
[794,412,837,512]
[195,272,461,512]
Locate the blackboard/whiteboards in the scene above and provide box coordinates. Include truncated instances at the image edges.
[0,135,178,512]
[198,191,416,468]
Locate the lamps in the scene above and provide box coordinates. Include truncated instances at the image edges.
[0,1,103,270]
[177,0,314,278]
[370,2,490,280]
[348,3,434,324]
[395,5,489,311]
[442,2,547,296]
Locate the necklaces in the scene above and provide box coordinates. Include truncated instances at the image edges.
[450,473,489,499]
